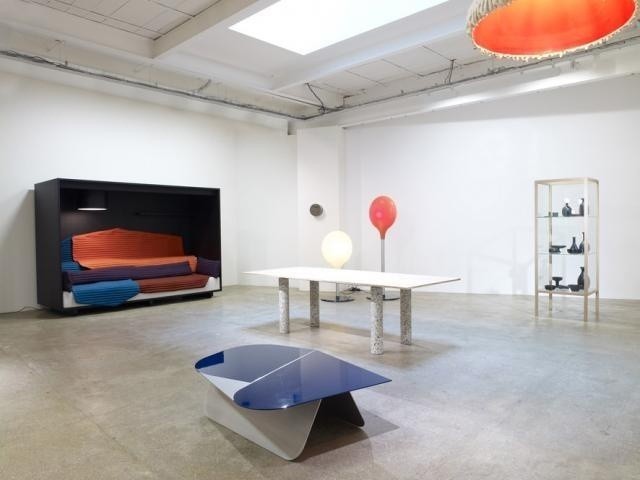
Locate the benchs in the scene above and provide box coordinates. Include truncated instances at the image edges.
[194,344,393,462]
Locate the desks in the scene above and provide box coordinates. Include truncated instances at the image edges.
[240,266,463,355]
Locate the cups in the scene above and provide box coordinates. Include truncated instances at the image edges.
[553,212,558,216]
[549,249,554,252]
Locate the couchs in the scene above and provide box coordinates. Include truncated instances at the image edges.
[60,228,221,312]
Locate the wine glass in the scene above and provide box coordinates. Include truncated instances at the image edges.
[552,276,562,288]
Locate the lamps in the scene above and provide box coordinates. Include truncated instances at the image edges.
[464,0,639,62]
[365,195,400,300]
[317,230,356,302]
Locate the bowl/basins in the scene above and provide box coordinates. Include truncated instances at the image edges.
[568,285,582,292]
[545,285,555,291]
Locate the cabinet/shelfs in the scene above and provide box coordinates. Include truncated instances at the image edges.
[534,178,600,323]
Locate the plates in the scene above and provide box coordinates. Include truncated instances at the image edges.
[567,249,582,253]
